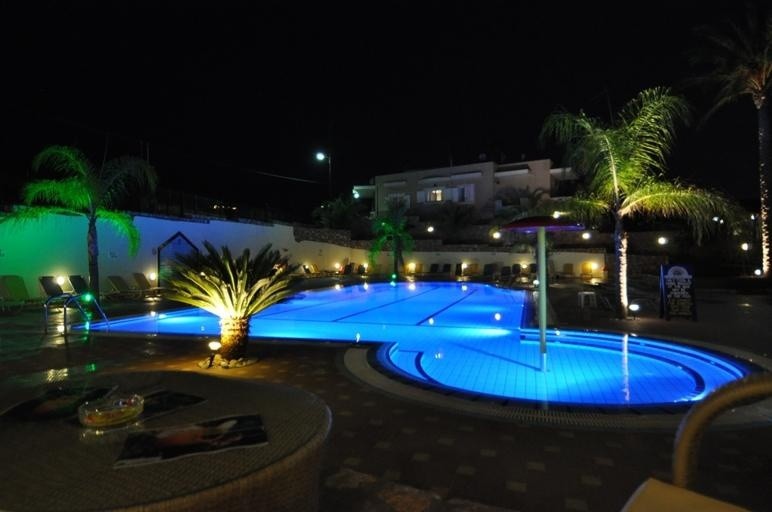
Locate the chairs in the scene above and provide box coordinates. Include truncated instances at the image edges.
[0,268,164,315]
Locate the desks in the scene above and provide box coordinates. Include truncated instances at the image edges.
[0,367,331,512]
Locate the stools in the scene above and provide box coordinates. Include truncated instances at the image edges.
[303,262,574,287]
[577,290,598,310]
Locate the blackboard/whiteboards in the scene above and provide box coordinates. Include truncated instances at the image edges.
[662,264,696,313]
